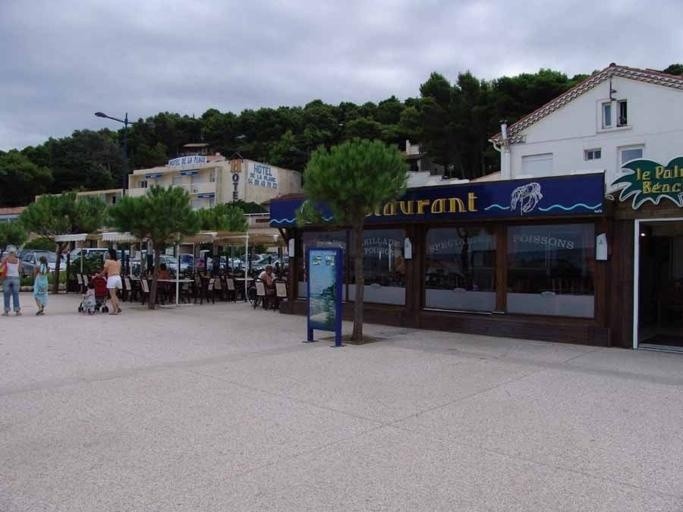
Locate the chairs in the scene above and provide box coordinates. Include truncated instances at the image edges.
[69,267,287,312]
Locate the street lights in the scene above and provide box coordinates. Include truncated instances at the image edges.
[94,111,157,188]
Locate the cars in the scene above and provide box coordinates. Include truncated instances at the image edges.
[0,244,288,278]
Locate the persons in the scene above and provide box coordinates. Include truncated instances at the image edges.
[95,247,122,315]
[159,263,170,279]
[32,255,50,314]
[256,264,280,310]
[0,246,22,316]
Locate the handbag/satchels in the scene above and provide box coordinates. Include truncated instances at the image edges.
[0,257,8,279]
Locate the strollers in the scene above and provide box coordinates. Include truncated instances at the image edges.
[77,274,110,314]
